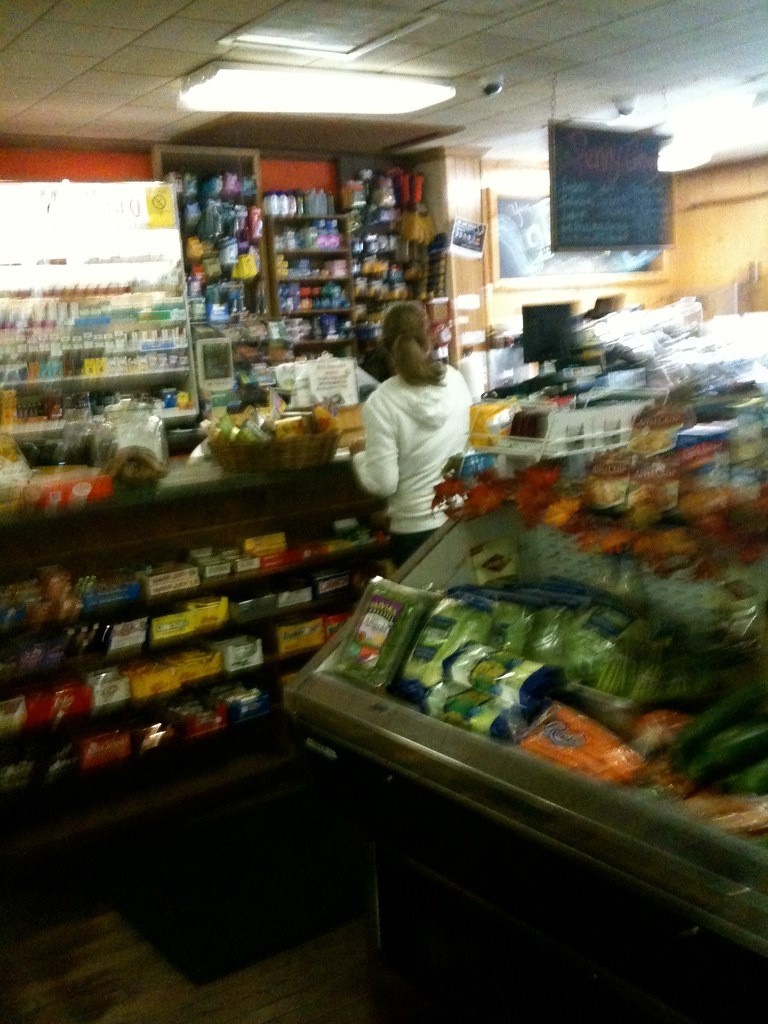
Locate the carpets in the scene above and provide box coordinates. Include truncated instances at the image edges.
[71,792,378,987]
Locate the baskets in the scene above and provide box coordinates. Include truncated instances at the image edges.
[207,426,342,471]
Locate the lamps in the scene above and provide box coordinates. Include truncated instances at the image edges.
[178,61,456,116]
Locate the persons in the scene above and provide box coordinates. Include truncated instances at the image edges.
[348,302,473,571]
[494,293,626,390]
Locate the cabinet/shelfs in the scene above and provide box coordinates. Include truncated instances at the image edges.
[1,530,392,859]
[0,181,200,442]
[264,213,358,360]
[277,489,768,1023]
[456,386,672,486]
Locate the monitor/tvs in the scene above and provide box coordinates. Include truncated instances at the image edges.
[521,302,575,363]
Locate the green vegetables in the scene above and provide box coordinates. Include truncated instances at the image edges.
[335,583,627,739]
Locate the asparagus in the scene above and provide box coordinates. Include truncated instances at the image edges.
[594,620,707,704]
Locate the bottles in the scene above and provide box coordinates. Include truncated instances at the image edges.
[0,165,450,449]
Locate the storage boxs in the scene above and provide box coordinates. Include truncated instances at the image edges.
[0,518,370,790]
[23,475,113,506]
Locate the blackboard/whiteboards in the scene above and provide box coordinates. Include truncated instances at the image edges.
[480,187,669,293]
[548,119,676,252]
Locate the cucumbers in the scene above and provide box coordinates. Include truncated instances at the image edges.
[664,676,768,792]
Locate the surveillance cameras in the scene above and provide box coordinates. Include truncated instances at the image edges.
[616,98,637,116]
[478,76,504,97]
